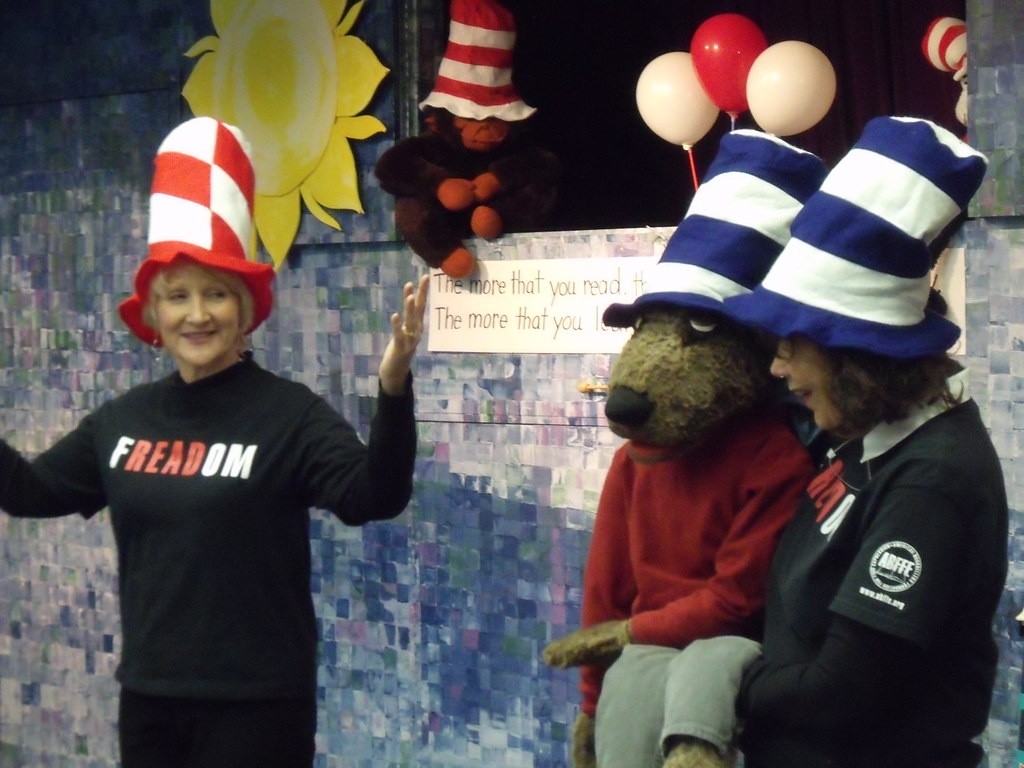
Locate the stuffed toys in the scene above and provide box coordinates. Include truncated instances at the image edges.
[920,17,969,144]
[542,128,821,768]
[375,0,537,278]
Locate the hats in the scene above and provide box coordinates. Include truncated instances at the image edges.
[725,114,989,359]
[117,117,274,345]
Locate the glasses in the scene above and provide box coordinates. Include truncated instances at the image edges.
[764,334,796,362]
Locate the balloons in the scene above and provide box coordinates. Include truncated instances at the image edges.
[745,40,836,138]
[690,14,767,121]
[635,53,720,151]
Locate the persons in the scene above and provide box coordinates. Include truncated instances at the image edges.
[737,117,1008,768]
[0,118,433,767]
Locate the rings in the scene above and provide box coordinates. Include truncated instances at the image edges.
[404,329,414,337]
